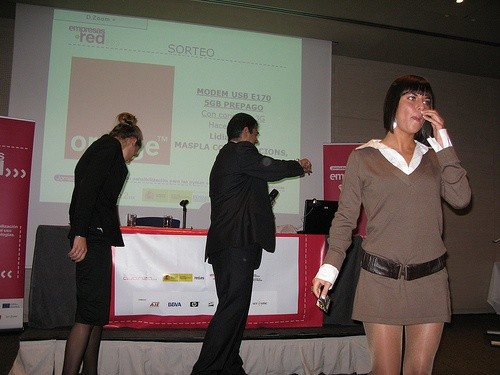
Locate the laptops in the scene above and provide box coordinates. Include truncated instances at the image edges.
[296,199,338,234]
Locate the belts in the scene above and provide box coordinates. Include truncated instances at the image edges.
[360,252,445,281]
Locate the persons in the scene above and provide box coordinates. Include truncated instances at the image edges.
[59,112,144,375]
[189,112,315,375]
[313,73,473,375]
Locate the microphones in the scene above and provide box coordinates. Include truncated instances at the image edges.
[269,189,280,201]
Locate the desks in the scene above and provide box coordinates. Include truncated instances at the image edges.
[28,225,363,328]
